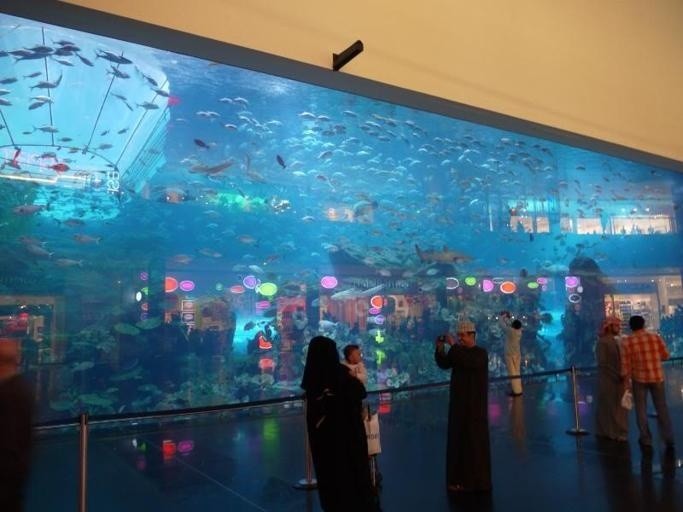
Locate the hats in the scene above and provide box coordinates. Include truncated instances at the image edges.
[456,320,475,333]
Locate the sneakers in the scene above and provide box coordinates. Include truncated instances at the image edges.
[607,433,675,447]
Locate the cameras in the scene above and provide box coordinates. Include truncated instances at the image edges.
[436,335,444,341]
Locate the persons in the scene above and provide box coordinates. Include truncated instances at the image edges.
[342,337,368,386]
[507,393,529,463]
[596,442,639,509]
[296,334,382,511]
[640,441,677,511]
[497,310,525,398]
[621,315,676,456]
[432,319,493,495]
[0,336,37,512]
[592,311,631,445]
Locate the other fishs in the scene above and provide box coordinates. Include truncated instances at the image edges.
[0,39,683,414]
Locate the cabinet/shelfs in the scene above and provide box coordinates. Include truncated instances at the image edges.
[619,303,632,334]
